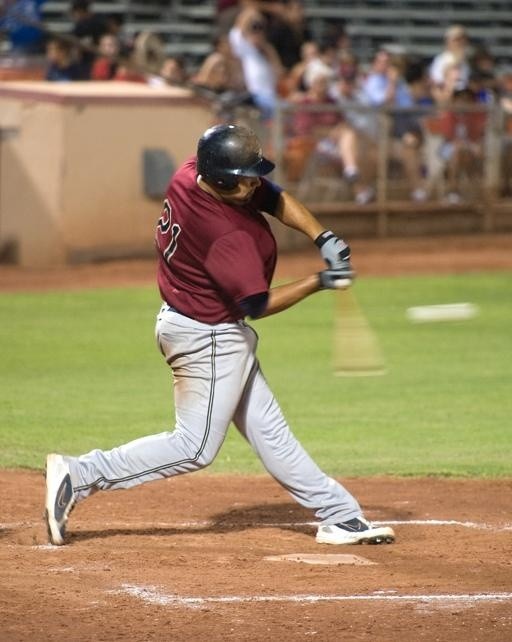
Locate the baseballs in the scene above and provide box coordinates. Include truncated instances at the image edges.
[405,300,473,325]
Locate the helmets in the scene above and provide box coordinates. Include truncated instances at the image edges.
[196,123,275,190]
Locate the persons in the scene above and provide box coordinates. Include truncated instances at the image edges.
[0,0,186,89]
[40,122,404,551]
[191,0,512,207]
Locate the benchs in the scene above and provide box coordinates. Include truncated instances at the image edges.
[41,0,512,180]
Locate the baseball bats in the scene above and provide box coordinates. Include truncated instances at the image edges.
[330,288,384,376]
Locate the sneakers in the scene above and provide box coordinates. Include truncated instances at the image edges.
[315,515,396,545]
[46,453,81,545]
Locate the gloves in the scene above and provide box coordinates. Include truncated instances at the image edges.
[314,230,356,290]
